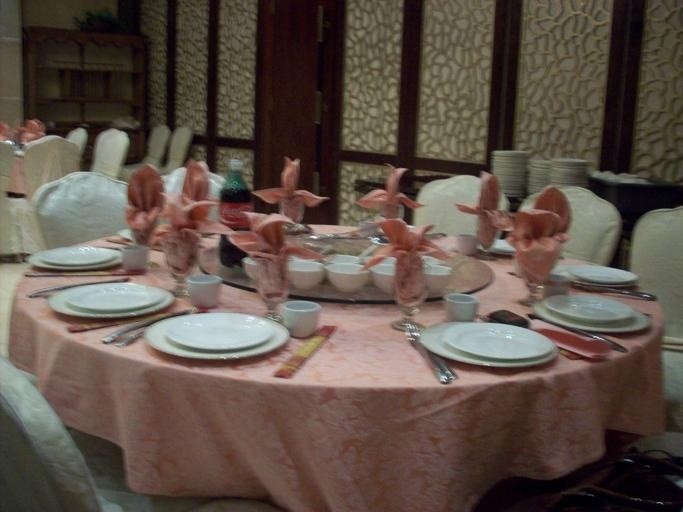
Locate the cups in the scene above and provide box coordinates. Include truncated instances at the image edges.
[377,202,405,221]
[327,263,369,293]
[256,260,293,322]
[444,294,478,322]
[180,206,209,238]
[324,255,361,263]
[359,222,377,238]
[424,265,452,294]
[366,255,398,265]
[163,235,201,286]
[279,197,305,233]
[456,234,478,256]
[186,275,223,308]
[282,301,321,338]
[371,264,396,294]
[422,256,440,266]
[129,218,157,246]
[121,245,150,272]
[242,257,258,279]
[288,261,324,289]
[476,213,497,249]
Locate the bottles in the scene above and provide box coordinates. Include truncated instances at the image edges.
[218,160,254,268]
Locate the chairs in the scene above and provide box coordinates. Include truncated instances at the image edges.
[67,127,88,152]
[18,134,81,263]
[0,359,208,512]
[1,140,22,263]
[157,126,195,175]
[413,173,509,245]
[629,207,682,432]
[123,125,170,173]
[91,127,130,178]
[515,183,622,267]
[161,168,228,227]
[30,170,135,249]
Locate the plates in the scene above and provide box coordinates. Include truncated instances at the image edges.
[542,295,632,324]
[166,318,274,350]
[480,244,516,255]
[28,248,122,269]
[548,158,590,188]
[65,283,166,311]
[526,159,551,194]
[144,312,290,359]
[533,301,650,332]
[552,264,638,287]
[490,239,519,252]
[443,324,554,359]
[490,150,529,198]
[39,247,117,265]
[49,287,174,318]
[118,228,132,240]
[419,321,559,367]
[567,266,638,283]
[303,234,371,252]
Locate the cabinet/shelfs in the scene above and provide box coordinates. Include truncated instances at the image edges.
[355,174,632,268]
[23,23,149,164]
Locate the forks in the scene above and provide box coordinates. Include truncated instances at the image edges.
[402,322,450,384]
